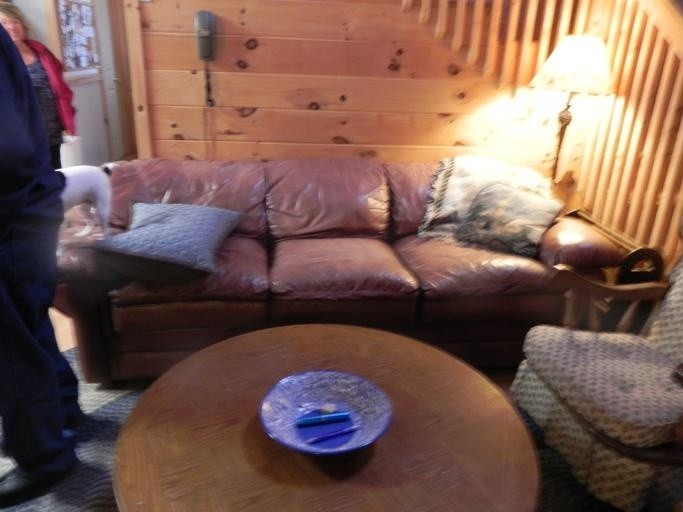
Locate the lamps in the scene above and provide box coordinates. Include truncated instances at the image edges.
[527,30,620,184]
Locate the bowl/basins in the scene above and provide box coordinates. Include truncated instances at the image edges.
[257,371,394,458]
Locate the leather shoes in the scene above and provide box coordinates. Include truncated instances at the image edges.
[0,458,81,510]
[0,411,85,451]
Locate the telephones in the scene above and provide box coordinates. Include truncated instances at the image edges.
[195,11,214,60]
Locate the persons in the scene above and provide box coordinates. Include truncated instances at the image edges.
[0,2,77,169]
[0,26,101,506]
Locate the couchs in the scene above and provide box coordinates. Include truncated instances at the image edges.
[46,158,632,383]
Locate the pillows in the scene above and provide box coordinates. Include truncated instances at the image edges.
[456,179,565,254]
[419,153,553,241]
[99,199,245,272]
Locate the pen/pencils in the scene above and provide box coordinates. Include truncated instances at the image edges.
[296,412,350,426]
[307,425,360,443]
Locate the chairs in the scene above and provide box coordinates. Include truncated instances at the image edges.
[512,253,682,512]
